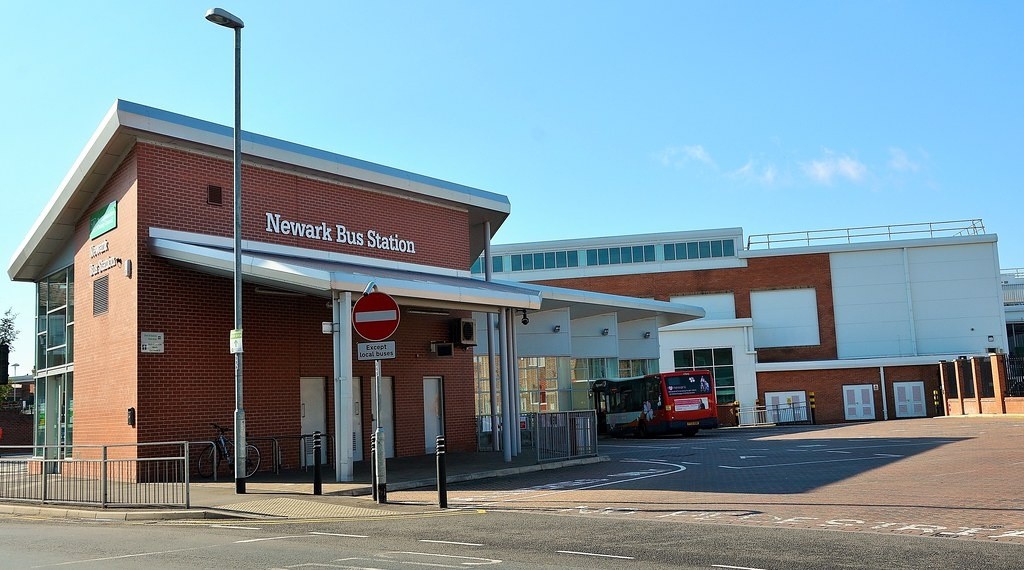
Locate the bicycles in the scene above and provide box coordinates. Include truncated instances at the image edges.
[198,422,261,479]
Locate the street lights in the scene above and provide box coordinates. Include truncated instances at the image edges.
[205,8,246,495]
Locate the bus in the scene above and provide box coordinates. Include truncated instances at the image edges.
[592,370,719,438]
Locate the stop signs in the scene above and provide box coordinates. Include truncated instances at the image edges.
[352,292,400,342]
